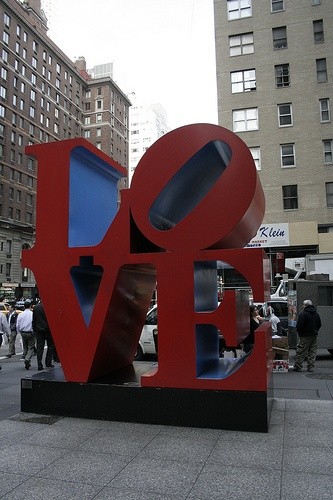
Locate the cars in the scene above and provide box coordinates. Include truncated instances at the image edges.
[132,304,158,361]
[0,299,25,317]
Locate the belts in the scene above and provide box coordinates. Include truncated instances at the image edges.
[21,331,32,333]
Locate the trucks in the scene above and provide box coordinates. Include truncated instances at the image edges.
[249,253,333,336]
[287,273,333,358]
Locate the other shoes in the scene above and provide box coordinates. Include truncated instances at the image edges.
[24,360,29,370]
[46,364,55,368]
[307,367,313,372]
[293,367,301,372]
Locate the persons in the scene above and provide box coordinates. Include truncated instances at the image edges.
[243,304,262,353]
[292,300,322,371]
[16,302,36,370]
[6,305,18,358]
[32,301,59,370]
[288,299,297,321]
[0,313,11,370]
[19,296,35,304]
[258,306,280,334]
